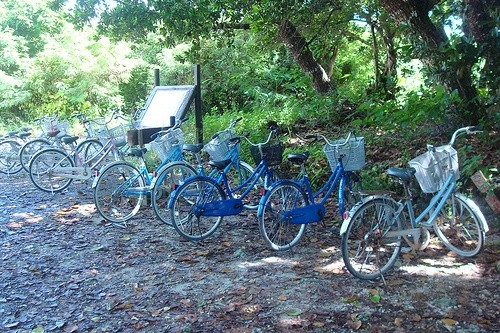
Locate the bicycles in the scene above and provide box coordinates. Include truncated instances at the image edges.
[339,125,491,287]
[0,108,146,197]
[92,118,201,229]
[167,119,291,248]
[148,116,264,232]
[257,129,367,258]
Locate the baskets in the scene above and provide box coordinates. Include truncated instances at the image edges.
[408,145,460,193]
[203,130,241,162]
[98,125,126,150]
[251,138,282,168]
[48,121,69,134]
[150,128,183,162]
[123,120,141,132]
[44,119,58,130]
[324,137,366,173]
[90,117,106,133]
